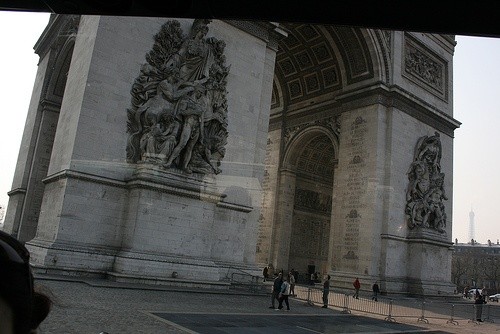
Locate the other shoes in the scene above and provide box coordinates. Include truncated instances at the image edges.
[269,307,275,309]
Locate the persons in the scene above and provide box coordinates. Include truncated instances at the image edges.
[311,272,320,286]
[407,163,448,228]
[418,137,439,173]
[476,289,479,298]
[372,281,380,302]
[166,24,214,89]
[465,288,469,298]
[273,277,290,311]
[321,276,329,308]
[0,229,51,334]
[289,269,299,295]
[263,267,269,282]
[353,277,360,299]
[156,66,194,101]
[269,273,284,309]
[163,85,204,174]
[482,288,487,302]
[474,295,483,322]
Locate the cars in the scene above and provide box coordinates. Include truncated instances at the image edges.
[489,294,500,302]
[464,288,483,297]
